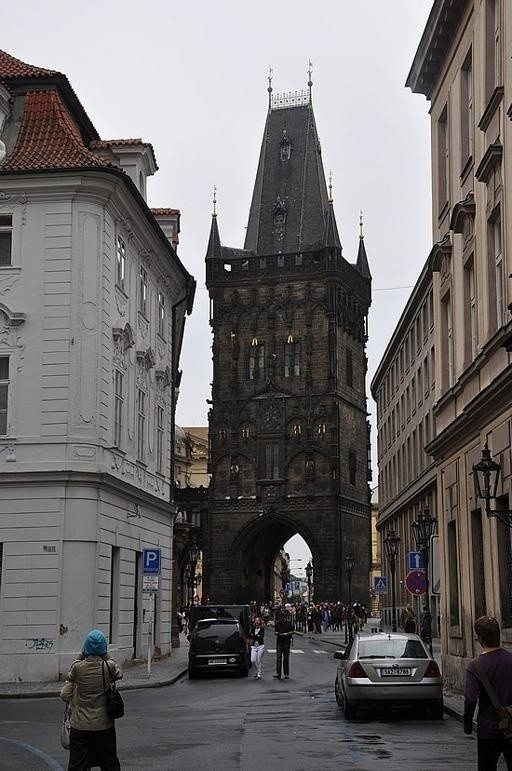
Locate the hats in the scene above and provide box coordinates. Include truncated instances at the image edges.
[82,630,107,656]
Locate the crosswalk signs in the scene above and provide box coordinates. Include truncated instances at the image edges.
[374,575,389,592]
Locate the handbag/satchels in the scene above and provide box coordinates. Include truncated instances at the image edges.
[497,704,512,738]
[61,719,71,750]
[106,684,124,719]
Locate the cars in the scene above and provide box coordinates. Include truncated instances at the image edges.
[187,616,252,682]
[331,630,445,723]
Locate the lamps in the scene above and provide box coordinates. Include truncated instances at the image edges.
[469,428,511,530]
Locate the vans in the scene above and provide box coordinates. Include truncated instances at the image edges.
[186,604,252,643]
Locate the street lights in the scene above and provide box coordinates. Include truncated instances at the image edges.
[342,550,356,646]
[289,557,303,576]
[188,534,201,609]
[381,529,402,632]
[409,508,438,658]
[304,561,315,607]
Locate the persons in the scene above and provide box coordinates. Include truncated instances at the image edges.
[58,629,126,771]
[464,616,511,771]
[177,600,440,681]
